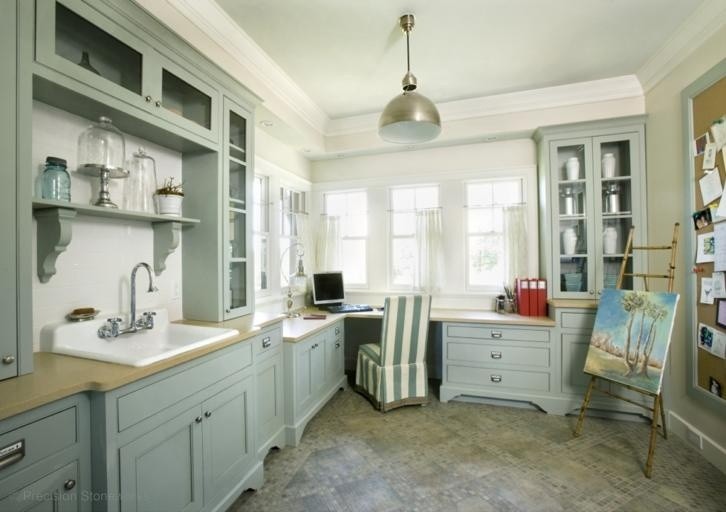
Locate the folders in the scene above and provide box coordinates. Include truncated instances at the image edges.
[516,278,547,316]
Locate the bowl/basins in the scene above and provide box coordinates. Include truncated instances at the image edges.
[68,311,99,321]
[565,273,626,291]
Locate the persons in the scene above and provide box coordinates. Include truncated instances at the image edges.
[701,328,711,345]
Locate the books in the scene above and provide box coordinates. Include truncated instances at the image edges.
[515,278,546,317]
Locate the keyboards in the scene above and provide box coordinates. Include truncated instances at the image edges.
[327,304,373,313]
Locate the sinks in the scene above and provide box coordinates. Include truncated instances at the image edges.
[50,324,240,366]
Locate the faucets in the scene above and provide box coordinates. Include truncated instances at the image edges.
[127,262,160,333]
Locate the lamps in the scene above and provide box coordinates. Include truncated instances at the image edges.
[275,239,308,319]
[377,12,441,148]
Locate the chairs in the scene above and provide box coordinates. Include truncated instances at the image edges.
[356,296,433,413]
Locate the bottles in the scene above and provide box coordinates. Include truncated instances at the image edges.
[41,156,71,202]
[563,152,621,253]
[495,296,515,313]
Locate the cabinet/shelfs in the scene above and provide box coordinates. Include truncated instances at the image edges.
[548,300,668,426]
[536,124,654,299]
[253,161,312,305]
[283,320,349,448]
[438,323,553,412]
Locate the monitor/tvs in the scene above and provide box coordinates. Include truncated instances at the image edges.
[311,271,346,311]
